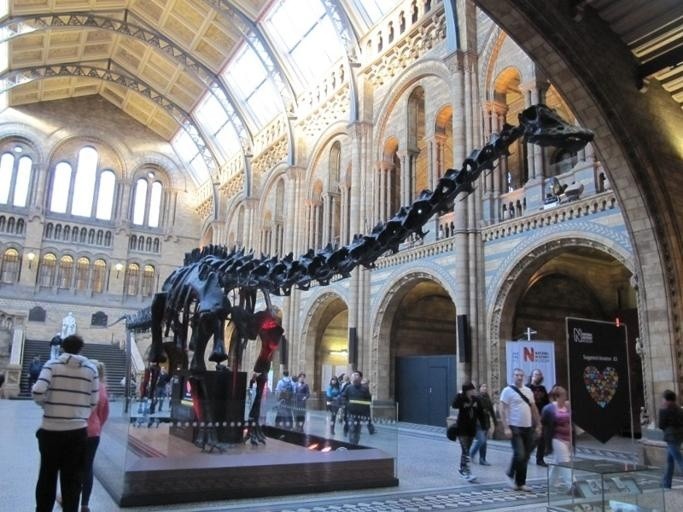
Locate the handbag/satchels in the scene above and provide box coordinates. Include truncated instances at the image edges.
[446,422,463,442]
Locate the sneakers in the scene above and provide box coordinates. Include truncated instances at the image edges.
[512,483,533,493]
[479,456,491,467]
[536,457,548,468]
[462,474,478,483]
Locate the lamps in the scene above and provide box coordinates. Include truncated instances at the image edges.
[547,176,564,205]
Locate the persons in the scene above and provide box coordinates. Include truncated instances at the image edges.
[657,389,683,488]
[541,385,577,490]
[470,383,497,466]
[49,332,62,360]
[249,370,311,432]
[325,370,372,445]
[451,382,487,482]
[523,368,548,467]
[31,334,99,512]
[27,354,41,393]
[62,311,75,339]
[498,368,543,491]
[56,358,110,512]
[135,366,172,415]
